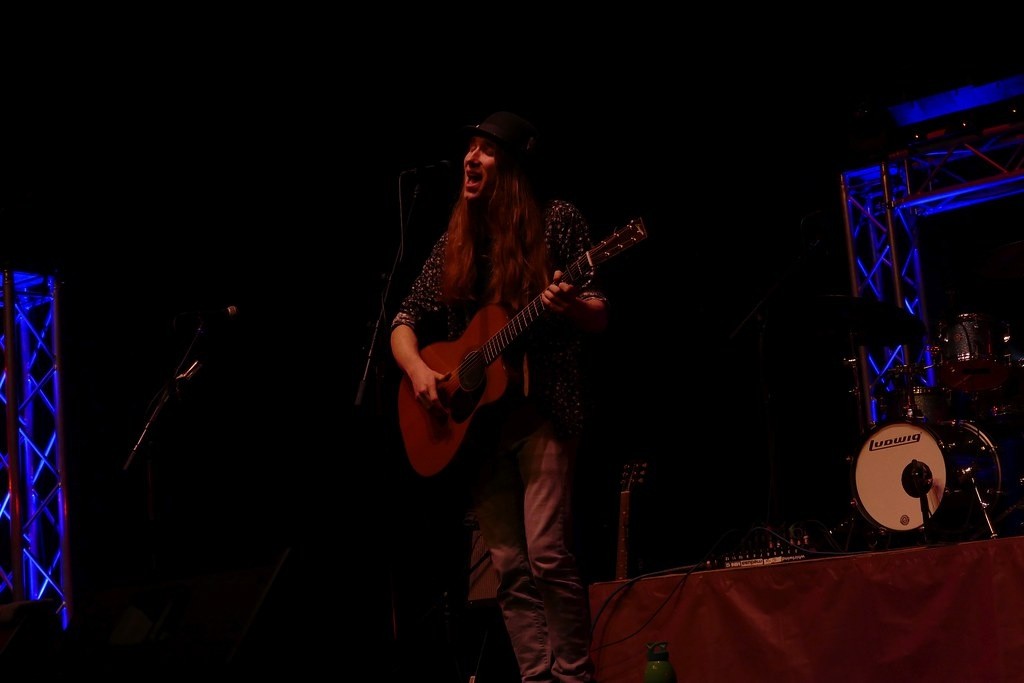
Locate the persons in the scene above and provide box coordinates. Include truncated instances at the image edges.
[390,111,609,683]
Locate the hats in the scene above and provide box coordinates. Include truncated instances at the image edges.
[463,111,539,166]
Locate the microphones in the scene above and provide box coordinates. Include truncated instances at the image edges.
[184,307,236,318]
[911,460,925,479]
[401,160,448,175]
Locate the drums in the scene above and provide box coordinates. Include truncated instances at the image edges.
[791,297,926,351]
[891,384,951,425]
[853,414,1001,538]
[930,308,1018,393]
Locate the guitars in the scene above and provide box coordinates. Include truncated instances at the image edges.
[396,214,649,477]
